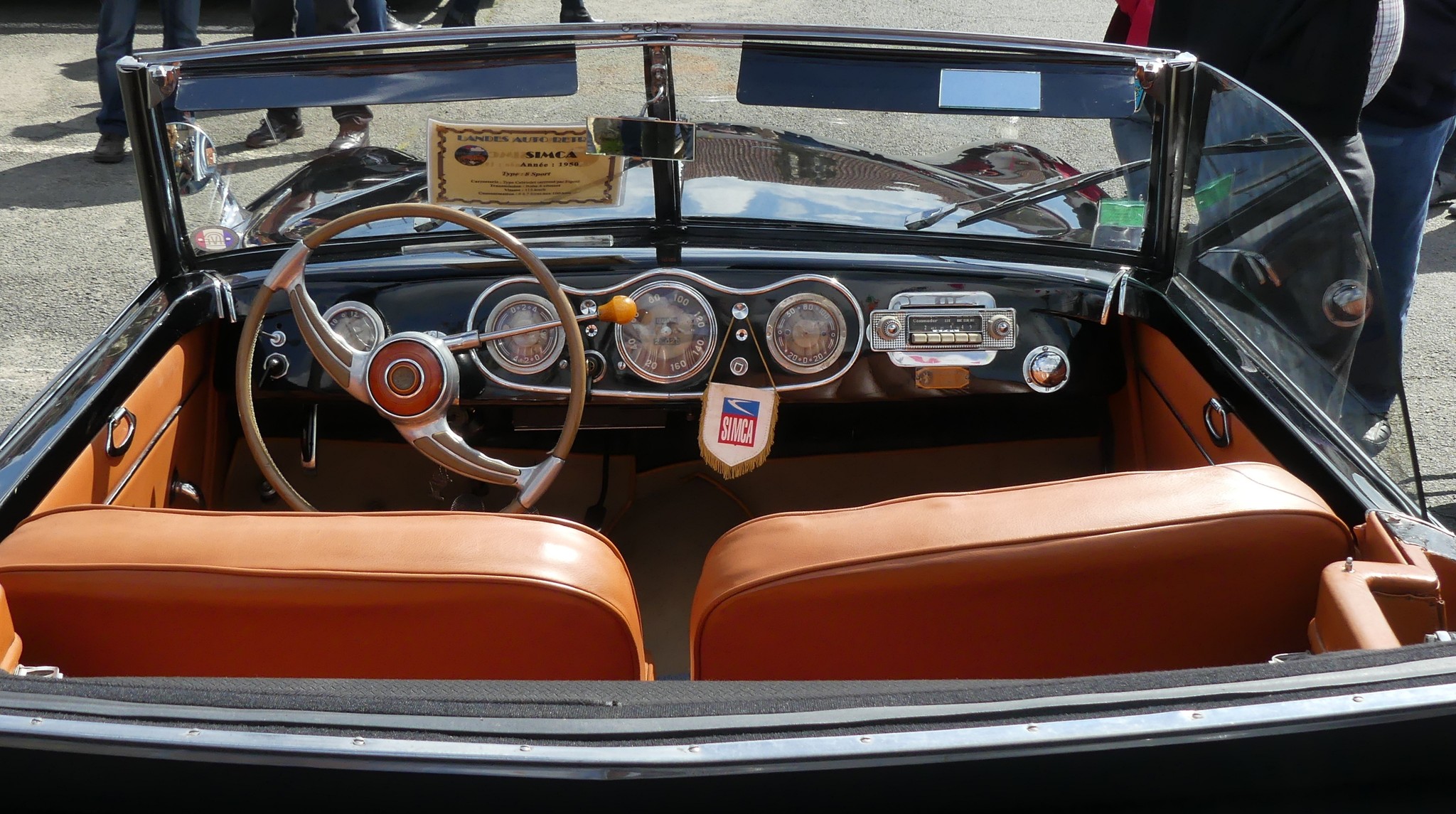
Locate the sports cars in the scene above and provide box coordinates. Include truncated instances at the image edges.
[0,17,1454,814]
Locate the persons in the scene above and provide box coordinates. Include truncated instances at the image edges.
[1341,0,1456,453]
[387,9,423,32]
[441,0,605,28]
[246,0,374,154]
[94,0,202,163]
[1102,1,1376,422]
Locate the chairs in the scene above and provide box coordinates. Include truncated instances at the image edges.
[1,507,662,682]
[690,463,1354,679]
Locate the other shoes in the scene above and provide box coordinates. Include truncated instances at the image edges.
[1428,170,1456,205]
[559,7,605,23]
[441,10,476,27]
[1448,202,1456,217]
[1340,411,1392,450]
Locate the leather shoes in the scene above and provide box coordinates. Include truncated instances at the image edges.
[328,122,370,152]
[246,117,305,148]
[94,133,126,162]
